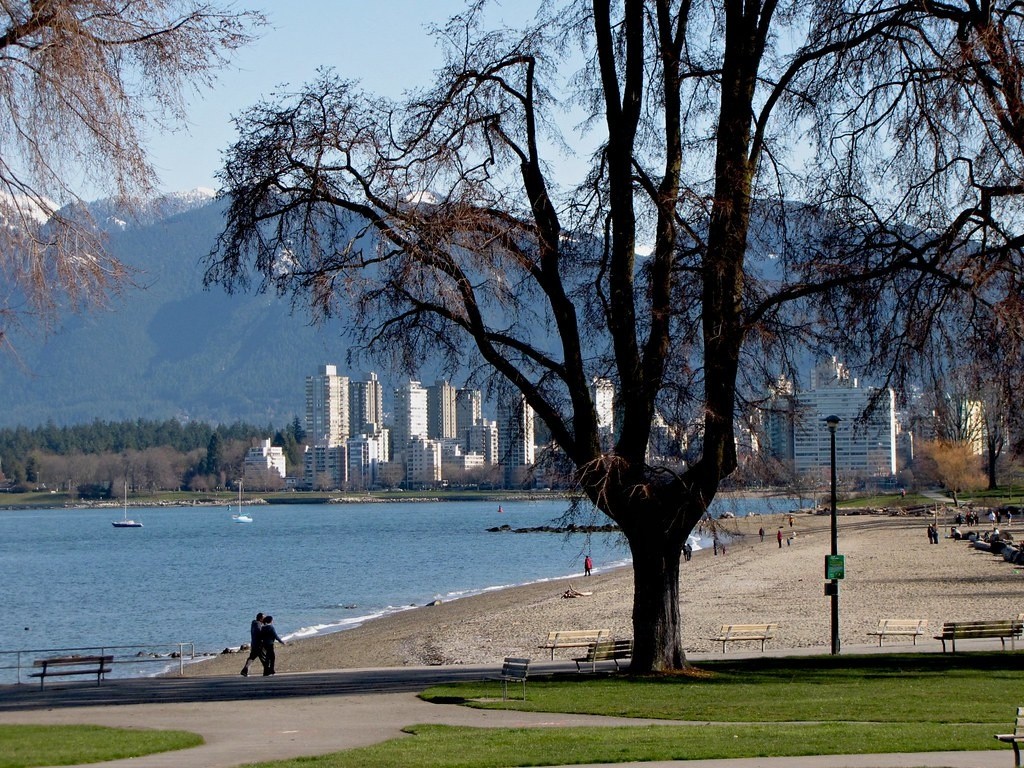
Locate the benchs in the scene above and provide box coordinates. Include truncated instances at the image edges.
[572,640,634,672]
[866,619,928,648]
[484,656,530,701]
[538,628,609,658]
[934,619,1023,651]
[710,623,778,654]
[31,655,114,690]
[994,706,1024,768]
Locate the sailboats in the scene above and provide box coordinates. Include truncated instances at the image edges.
[232,479,252,523]
[111,480,144,528]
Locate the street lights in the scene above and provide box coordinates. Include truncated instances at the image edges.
[819,416,849,657]
[865,434,870,481]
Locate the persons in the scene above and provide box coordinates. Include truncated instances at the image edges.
[261,616,286,677]
[927,507,1024,564]
[585,556,592,576]
[790,532,796,539]
[714,540,717,555]
[787,538,791,546]
[241,613,270,677]
[723,547,726,554]
[902,489,905,497]
[790,516,792,527]
[683,545,687,560]
[687,544,692,561]
[777,530,782,548]
[759,527,764,542]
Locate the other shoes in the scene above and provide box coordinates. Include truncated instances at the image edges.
[240,672,250,677]
